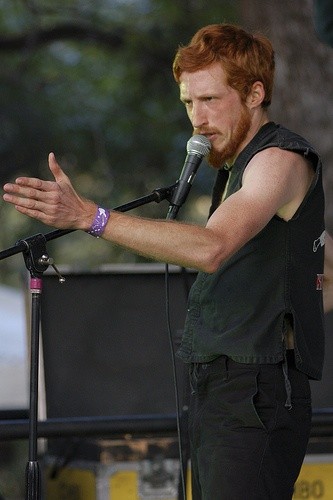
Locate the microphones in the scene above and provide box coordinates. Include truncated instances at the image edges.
[164,135,212,220]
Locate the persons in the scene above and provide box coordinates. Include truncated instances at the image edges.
[0,22,326,500]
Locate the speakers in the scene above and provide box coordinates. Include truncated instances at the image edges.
[41,264,210,419]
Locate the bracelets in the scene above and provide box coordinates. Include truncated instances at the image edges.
[84,200,111,239]
